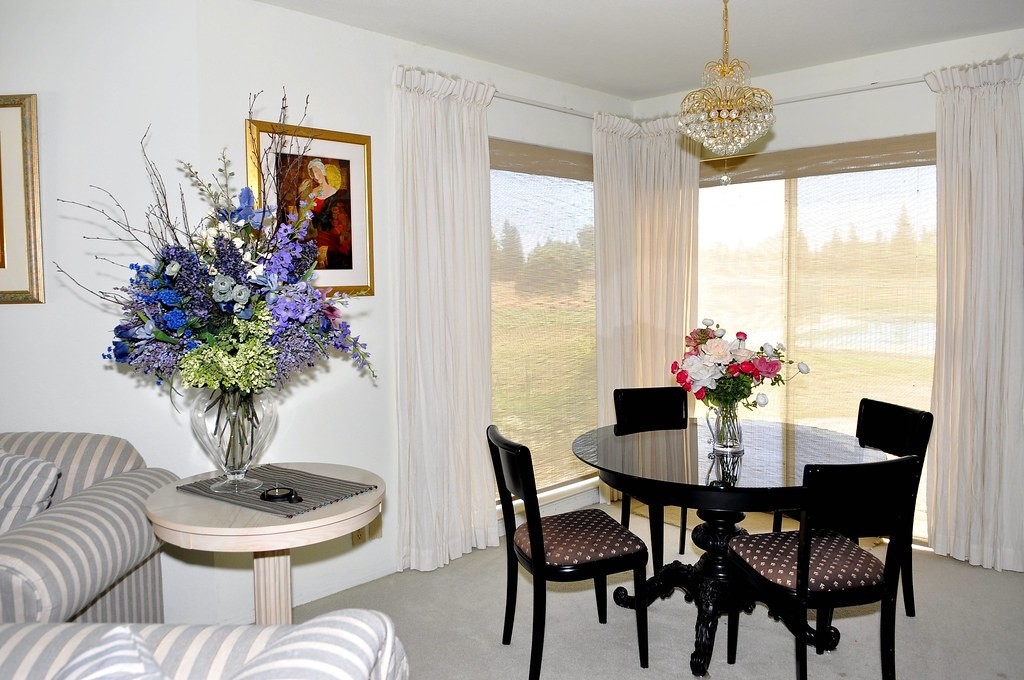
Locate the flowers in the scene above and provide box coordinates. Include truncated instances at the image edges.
[48,85,379,472]
[669,319,810,443]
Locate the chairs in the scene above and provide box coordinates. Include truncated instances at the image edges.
[486,424,649,680]
[612,387,692,554]
[770,397,935,617]
[725,455,920,680]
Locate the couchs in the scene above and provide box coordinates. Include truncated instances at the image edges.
[0,605,410,680]
[1,432,183,627]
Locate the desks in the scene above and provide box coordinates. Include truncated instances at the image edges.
[572,417,886,677]
[144,461,387,626]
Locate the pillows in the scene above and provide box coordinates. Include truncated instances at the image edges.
[0,442,61,534]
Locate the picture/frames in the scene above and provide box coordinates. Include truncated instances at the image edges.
[244,119,376,299]
[0,93,46,305]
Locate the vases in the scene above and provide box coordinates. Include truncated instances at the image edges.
[705,399,746,457]
[190,381,279,494]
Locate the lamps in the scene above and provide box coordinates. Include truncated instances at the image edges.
[676,0,775,185]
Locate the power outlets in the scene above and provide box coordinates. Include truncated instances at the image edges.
[352,527,367,548]
